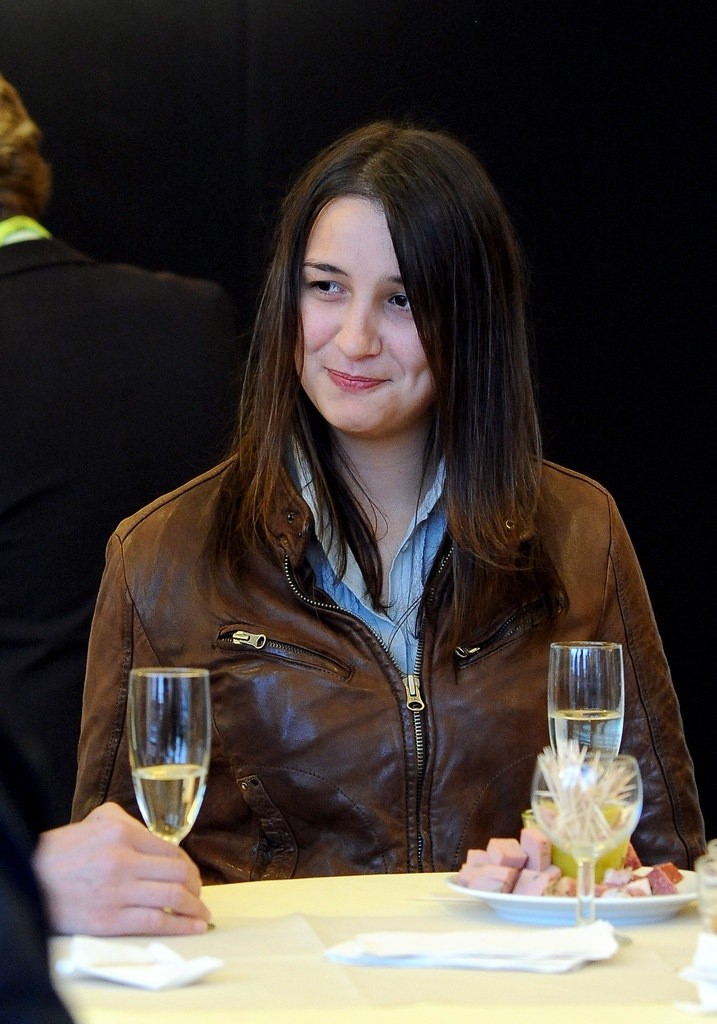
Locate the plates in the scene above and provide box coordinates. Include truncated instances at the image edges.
[444,875,694,927]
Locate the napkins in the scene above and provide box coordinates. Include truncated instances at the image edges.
[674,931,717,1016]
[323,919,618,976]
[55,933,224,992]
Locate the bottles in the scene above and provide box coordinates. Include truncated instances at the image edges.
[693,839,717,1011]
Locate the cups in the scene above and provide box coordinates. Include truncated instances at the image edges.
[126,667,210,850]
[546,640,627,760]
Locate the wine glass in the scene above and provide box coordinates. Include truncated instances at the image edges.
[531,752,644,952]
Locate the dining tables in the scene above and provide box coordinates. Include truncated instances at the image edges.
[50,865,717,1024]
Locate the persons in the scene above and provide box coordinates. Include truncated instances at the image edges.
[0,77,249,831]
[0,801,214,1024]
[68,116,709,885]
